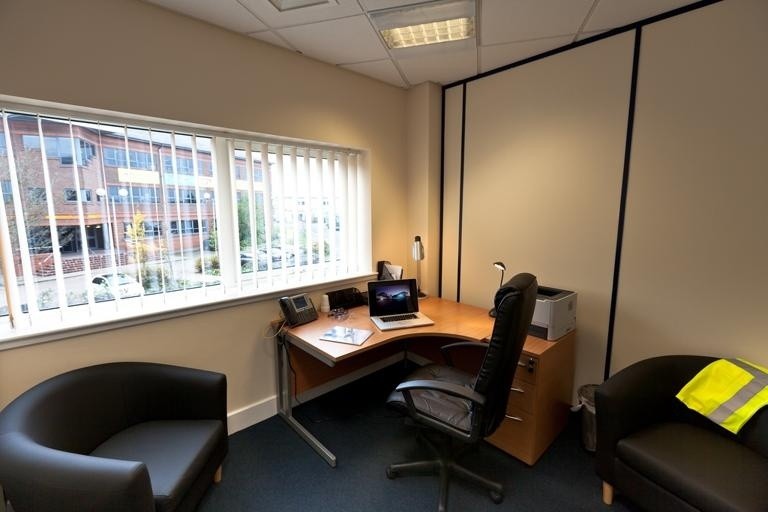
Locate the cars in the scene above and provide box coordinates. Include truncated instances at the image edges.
[239,246,295,270]
[86,271,144,303]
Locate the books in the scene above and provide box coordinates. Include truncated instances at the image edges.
[320,324,374,347]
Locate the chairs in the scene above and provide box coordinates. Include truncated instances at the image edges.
[592,351,768,511]
[0,359,232,511]
[382,269,540,512]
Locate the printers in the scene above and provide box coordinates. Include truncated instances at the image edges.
[528,286,578,341]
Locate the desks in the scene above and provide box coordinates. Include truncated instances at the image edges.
[271,292,577,469]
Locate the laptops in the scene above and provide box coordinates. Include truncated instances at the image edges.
[367,279,435,332]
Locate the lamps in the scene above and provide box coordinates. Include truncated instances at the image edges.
[488,260,507,318]
[412,235,428,300]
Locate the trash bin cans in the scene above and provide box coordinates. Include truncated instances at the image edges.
[577,384,599,452]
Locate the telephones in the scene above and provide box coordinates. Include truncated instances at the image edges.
[279,293,318,329]
